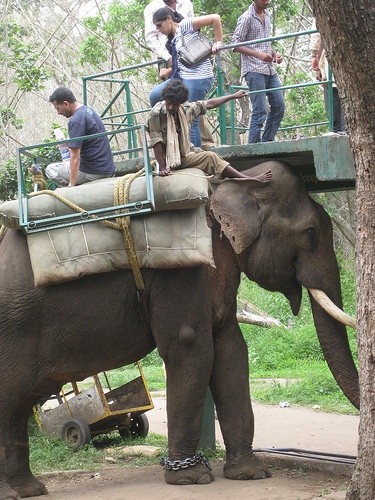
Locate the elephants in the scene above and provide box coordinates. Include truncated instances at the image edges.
[0,159,362,500]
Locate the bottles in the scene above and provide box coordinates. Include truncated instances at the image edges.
[55,128,71,161]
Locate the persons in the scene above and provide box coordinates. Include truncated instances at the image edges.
[144,0,215,149]
[149,8,224,146]
[144,80,273,181]
[45,87,115,188]
[232,0,285,143]
[312,18,347,135]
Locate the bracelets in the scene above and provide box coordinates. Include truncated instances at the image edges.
[313,55,320,58]
[68,186,71,188]
